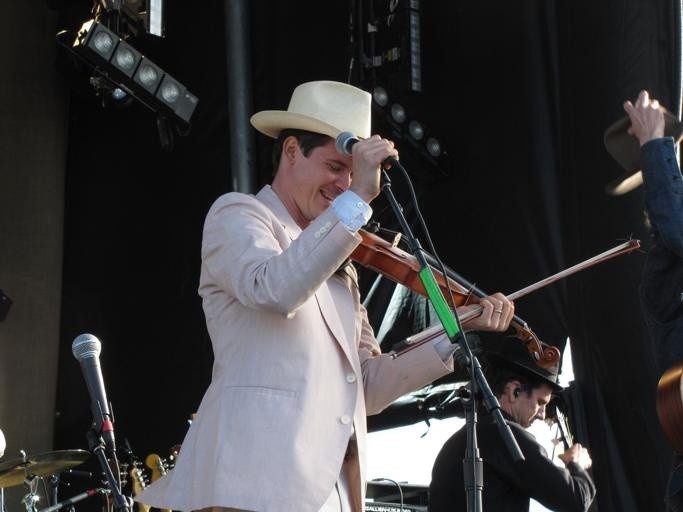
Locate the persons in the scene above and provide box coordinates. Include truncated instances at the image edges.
[623,88,682,512]
[428,341,598,512]
[131,80,516,511]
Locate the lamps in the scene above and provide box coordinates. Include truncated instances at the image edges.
[364,81,444,181]
[51,0,199,135]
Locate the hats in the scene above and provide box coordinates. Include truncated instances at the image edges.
[486,336,565,391]
[603,107,682,196]
[250,80,373,142]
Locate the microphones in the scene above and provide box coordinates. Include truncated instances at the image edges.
[335,131,398,168]
[72,333,117,454]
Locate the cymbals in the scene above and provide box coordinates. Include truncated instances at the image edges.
[0,449,87,487]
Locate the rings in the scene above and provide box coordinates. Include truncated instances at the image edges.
[494,310,502,313]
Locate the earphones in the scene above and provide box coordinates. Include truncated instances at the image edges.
[514,388,520,398]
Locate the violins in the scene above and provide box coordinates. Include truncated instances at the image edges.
[350,228,562,369]
